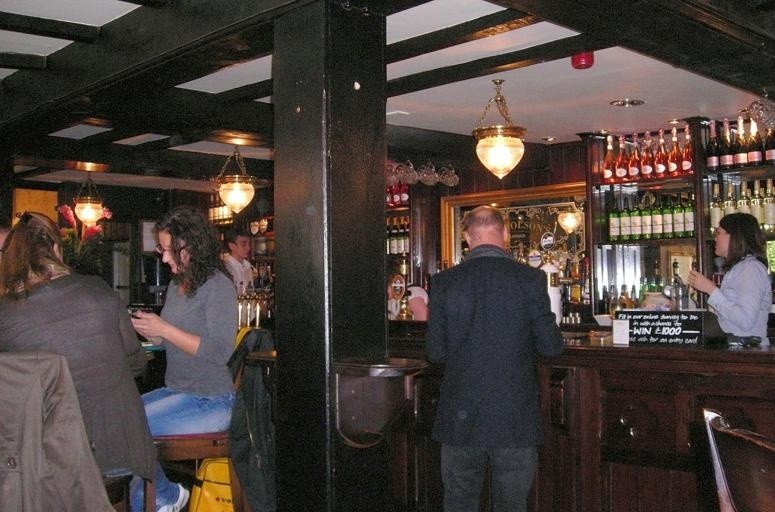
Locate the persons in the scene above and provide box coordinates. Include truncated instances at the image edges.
[1,210,155,482]
[388,261,429,322]
[686,212,773,346]
[221,226,259,325]
[425,205,566,511]
[127,205,239,511]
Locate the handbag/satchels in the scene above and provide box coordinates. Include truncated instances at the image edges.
[188,458,235,511]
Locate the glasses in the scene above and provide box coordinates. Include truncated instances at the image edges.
[154,243,190,257]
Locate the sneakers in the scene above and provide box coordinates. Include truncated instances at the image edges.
[157,483,190,511]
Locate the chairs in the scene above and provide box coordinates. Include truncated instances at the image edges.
[0,349,132,511]
[144,329,266,512]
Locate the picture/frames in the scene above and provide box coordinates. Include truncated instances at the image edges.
[440,182,586,307]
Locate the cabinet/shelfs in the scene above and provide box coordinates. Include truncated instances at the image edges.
[246,216,275,300]
[697,116,775,324]
[387,184,412,295]
[587,127,700,336]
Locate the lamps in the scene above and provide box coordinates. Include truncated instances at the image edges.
[72,170,104,228]
[212,144,256,214]
[471,79,526,182]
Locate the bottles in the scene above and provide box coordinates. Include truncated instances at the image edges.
[206,192,235,226]
[514,242,590,330]
[237,261,274,295]
[602,114,775,320]
[385,180,412,320]
